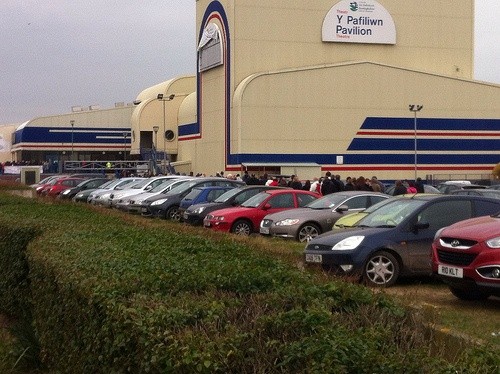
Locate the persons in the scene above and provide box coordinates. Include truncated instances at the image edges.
[216,171,241,180]
[139,172,162,178]
[189,172,206,177]
[0,160,58,174]
[242,171,311,191]
[310,172,385,196]
[106,162,111,168]
[393,177,424,196]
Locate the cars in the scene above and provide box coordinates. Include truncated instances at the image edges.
[28,174,206,215]
[302,194,500,288]
[180,185,237,215]
[139,177,247,223]
[379,180,500,200]
[259,190,392,244]
[202,189,322,237]
[331,191,430,231]
[183,185,295,226]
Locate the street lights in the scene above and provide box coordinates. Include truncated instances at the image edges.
[156,94,176,174]
[122,131,128,177]
[408,104,424,183]
[69,120,75,176]
[153,126,160,150]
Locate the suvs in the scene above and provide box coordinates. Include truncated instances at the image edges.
[430,213,500,301]
